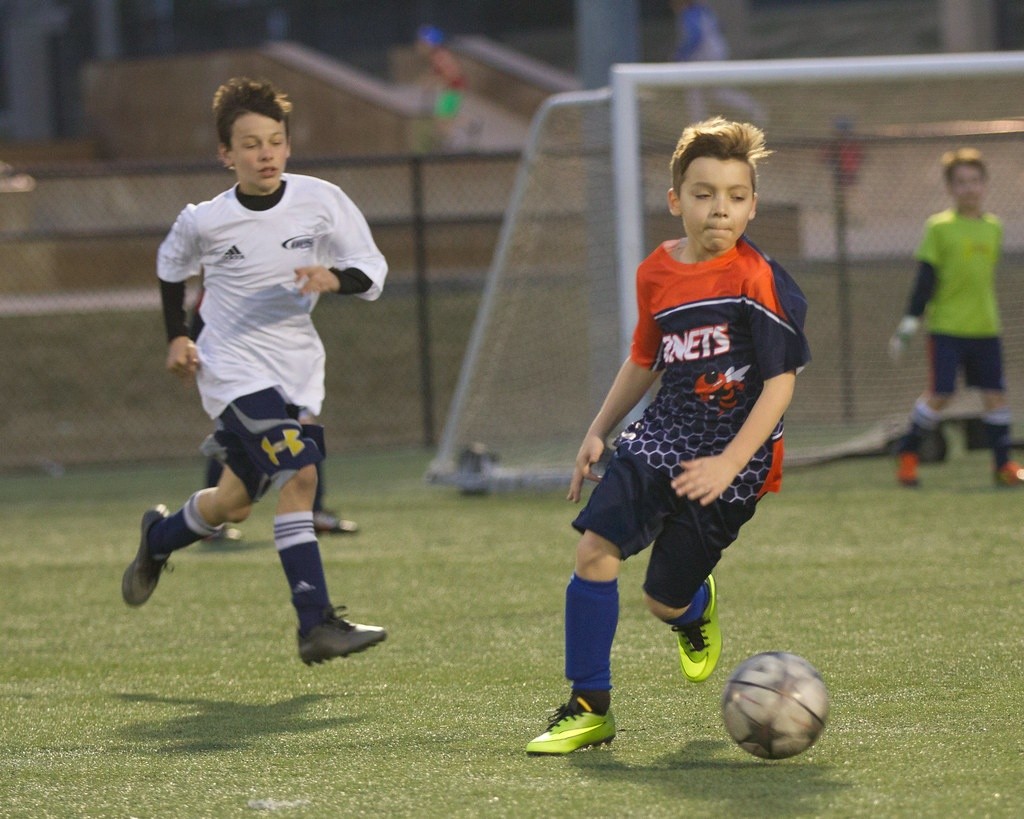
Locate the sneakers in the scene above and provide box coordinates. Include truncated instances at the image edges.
[123,504,171,606]
[671,573,721,684]
[526,702,618,756]
[296,604,387,665]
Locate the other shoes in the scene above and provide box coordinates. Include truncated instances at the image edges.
[898,438,920,485]
[314,511,358,534]
[202,522,241,541]
[994,461,1024,484]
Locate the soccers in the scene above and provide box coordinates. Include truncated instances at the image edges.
[722,650,829,759]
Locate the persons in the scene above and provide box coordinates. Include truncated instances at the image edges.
[525,116,813,753]
[886,147,1024,487]
[120,77,388,665]
[191,269,361,547]
